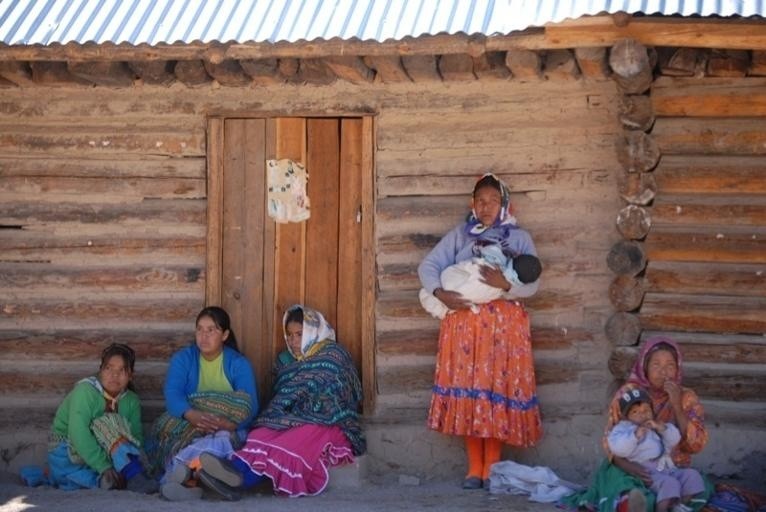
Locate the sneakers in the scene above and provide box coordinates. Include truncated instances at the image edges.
[167,463,192,487]
[196,467,242,503]
[127,472,160,495]
[162,481,205,503]
[627,487,647,512]
[482,478,492,490]
[198,449,243,488]
[462,475,482,489]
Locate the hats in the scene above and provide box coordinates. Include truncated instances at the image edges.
[618,388,654,417]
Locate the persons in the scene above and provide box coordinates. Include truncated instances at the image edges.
[46,342,161,495]
[582,335,717,512]
[154,305,260,502]
[193,303,369,502]
[415,172,541,491]
[606,388,707,512]
[418,241,543,321]
